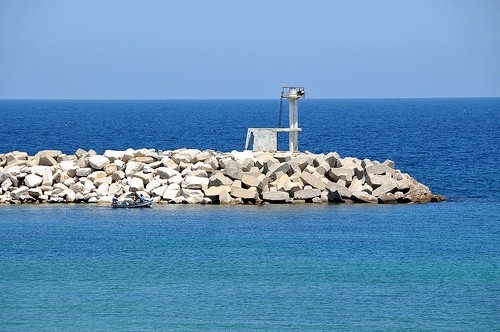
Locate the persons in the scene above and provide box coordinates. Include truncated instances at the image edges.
[112,194,118,205]
[130,197,137,205]
[139,194,145,202]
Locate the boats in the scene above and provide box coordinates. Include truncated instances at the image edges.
[111,199,154,207]
